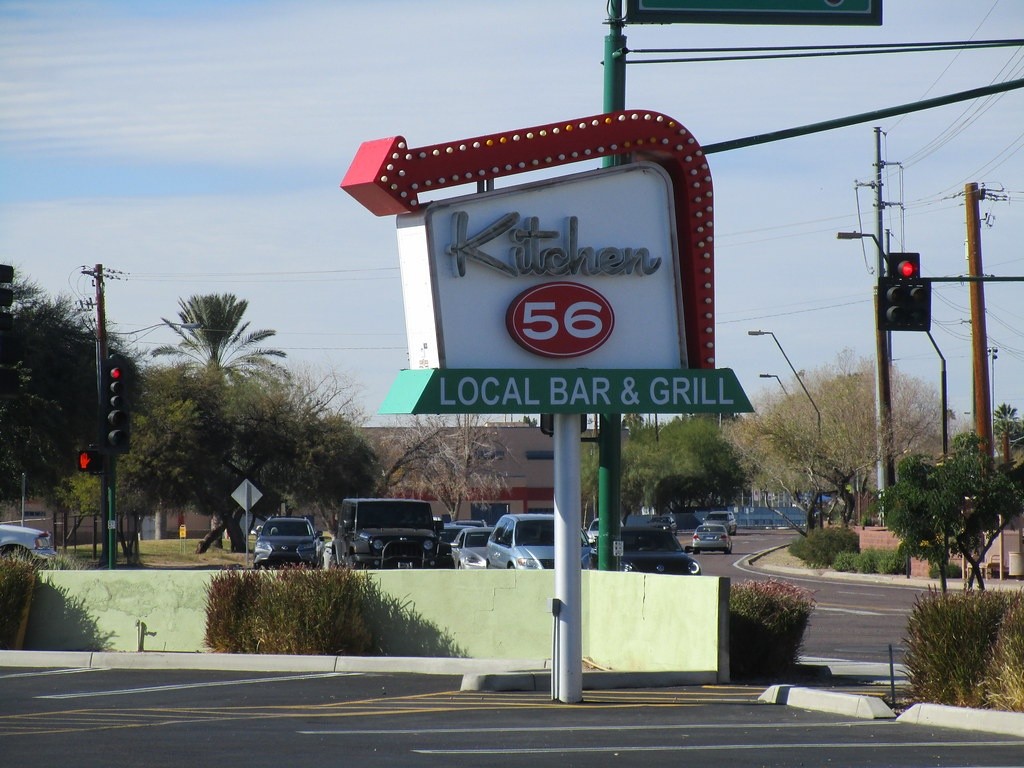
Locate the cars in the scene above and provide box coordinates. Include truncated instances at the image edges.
[0,526,56,567]
[691,524,733,555]
[486,514,596,570]
[448,527,495,569]
[647,515,679,537]
[437,517,485,560]
[589,524,702,575]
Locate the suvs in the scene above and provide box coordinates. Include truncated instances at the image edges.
[251,515,323,568]
[702,511,737,536]
[336,498,452,570]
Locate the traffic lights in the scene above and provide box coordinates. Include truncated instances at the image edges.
[877,253,931,331]
[78,451,100,472]
[0,263,13,331]
[96,354,130,455]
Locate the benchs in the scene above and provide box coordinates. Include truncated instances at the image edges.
[967,554,1000,581]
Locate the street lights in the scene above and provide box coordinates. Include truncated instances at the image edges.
[759,374,792,404]
[837,230,950,571]
[748,330,824,532]
